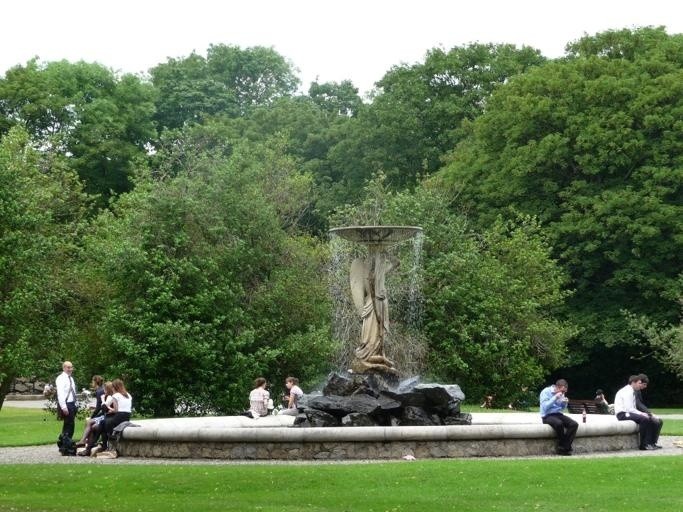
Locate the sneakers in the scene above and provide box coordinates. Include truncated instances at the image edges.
[75,441,90,456]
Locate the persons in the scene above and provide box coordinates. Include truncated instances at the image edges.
[74,381,115,448]
[348,243,400,377]
[594,389,614,414]
[77,379,132,456]
[538,378,578,455]
[614,375,657,450]
[284,376,303,409]
[635,372,663,448]
[55,360,77,437]
[85,374,105,421]
[248,378,269,418]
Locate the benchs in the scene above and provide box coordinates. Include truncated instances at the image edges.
[566,399,615,414]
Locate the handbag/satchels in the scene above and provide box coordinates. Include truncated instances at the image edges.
[56,432,77,455]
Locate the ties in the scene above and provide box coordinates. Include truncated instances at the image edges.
[68,376,77,401]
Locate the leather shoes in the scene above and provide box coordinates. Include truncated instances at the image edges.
[640,444,661,450]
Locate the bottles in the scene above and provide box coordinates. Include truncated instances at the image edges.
[582,408,587,423]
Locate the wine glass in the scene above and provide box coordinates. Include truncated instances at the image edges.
[280,393,286,401]
